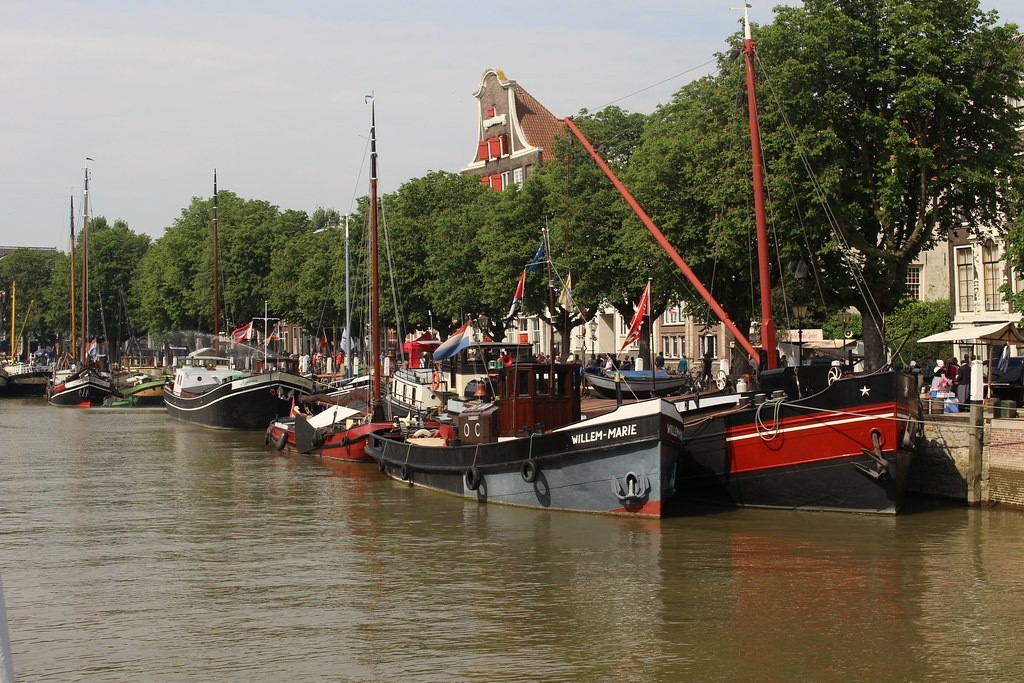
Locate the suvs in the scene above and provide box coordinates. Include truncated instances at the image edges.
[952,356,1024,407]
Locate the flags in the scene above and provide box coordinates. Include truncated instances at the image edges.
[341,329,354,352]
[319,333,328,350]
[87,340,96,355]
[433,321,471,361]
[267,324,280,346]
[509,272,524,317]
[617,286,650,359]
[528,242,544,272]
[559,277,573,312]
[232,323,252,341]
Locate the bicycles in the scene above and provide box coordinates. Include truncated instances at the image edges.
[679,367,703,394]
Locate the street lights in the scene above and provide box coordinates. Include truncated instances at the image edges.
[589,317,598,354]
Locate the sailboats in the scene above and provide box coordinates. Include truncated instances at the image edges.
[267,90,408,465]
[563,0,924,518]
[163,170,338,430]
[50,165,174,409]
[0,278,54,398]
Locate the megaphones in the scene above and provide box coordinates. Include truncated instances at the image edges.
[844,329,853,338]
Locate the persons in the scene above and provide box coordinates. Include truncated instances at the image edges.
[532,352,561,363]
[702,354,713,379]
[910,354,976,402]
[678,355,688,373]
[654,352,664,368]
[575,352,635,375]
[781,355,788,368]
[283,350,394,378]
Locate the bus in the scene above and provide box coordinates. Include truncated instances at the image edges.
[135,347,189,367]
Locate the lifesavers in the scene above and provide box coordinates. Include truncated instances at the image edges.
[432,371,440,390]
[465,466,481,490]
[521,460,539,482]
[277,432,287,450]
[311,437,317,446]
[264,431,272,446]
[378,458,385,472]
[340,436,348,446]
[400,464,410,480]
[412,428,431,438]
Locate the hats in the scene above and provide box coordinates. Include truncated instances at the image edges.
[937,359,943,366]
[910,361,916,366]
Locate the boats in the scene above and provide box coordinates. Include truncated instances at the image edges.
[364,312,697,518]
[583,368,690,399]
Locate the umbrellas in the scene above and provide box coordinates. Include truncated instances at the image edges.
[36,349,55,358]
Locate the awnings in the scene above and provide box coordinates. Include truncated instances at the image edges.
[916,321,1024,398]
